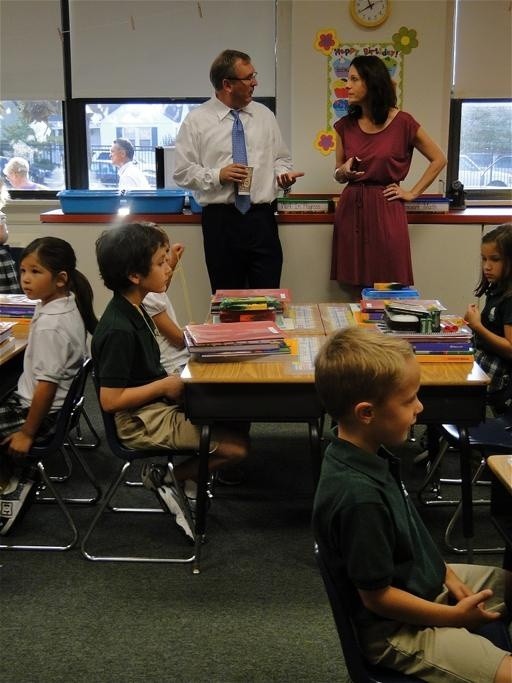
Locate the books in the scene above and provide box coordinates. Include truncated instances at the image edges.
[0,293,42,357]
[357,298,477,364]
[180,283,297,365]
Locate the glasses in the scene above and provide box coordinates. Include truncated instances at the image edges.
[229,71,257,85]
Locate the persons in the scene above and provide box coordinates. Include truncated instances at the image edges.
[0,235,100,536]
[107,136,151,191]
[139,219,192,375]
[3,156,51,189]
[309,323,511,681]
[172,47,305,294]
[463,220,512,399]
[88,219,251,546]
[0,208,29,293]
[328,53,448,301]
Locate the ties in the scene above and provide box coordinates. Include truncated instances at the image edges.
[227,109,252,215]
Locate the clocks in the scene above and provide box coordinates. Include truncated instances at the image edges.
[348,0,394,29]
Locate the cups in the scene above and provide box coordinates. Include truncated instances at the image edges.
[470,569,511,615]
[238,166,254,196]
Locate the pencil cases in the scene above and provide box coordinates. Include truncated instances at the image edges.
[218,296,280,323]
[360,288,420,309]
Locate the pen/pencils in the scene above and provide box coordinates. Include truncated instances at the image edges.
[485,601,508,615]
[394,302,418,306]
[362,309,384,312]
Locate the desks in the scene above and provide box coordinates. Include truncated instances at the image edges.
[0,302,85,441]
[176,302,328,575]
[317,297,493,539]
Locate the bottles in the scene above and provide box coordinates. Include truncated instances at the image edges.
[431,311,441,332]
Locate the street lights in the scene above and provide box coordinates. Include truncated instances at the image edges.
[484,146,493,183]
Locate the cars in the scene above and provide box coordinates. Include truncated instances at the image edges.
[90,149,156,183]
[458,151,512,189]
[0,154,54,186]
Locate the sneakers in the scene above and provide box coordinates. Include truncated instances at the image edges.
[156,478,198,544]
[0,477,34,536]
[139,460,177,493]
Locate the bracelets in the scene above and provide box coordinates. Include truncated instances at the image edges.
[332,167,348,184]
